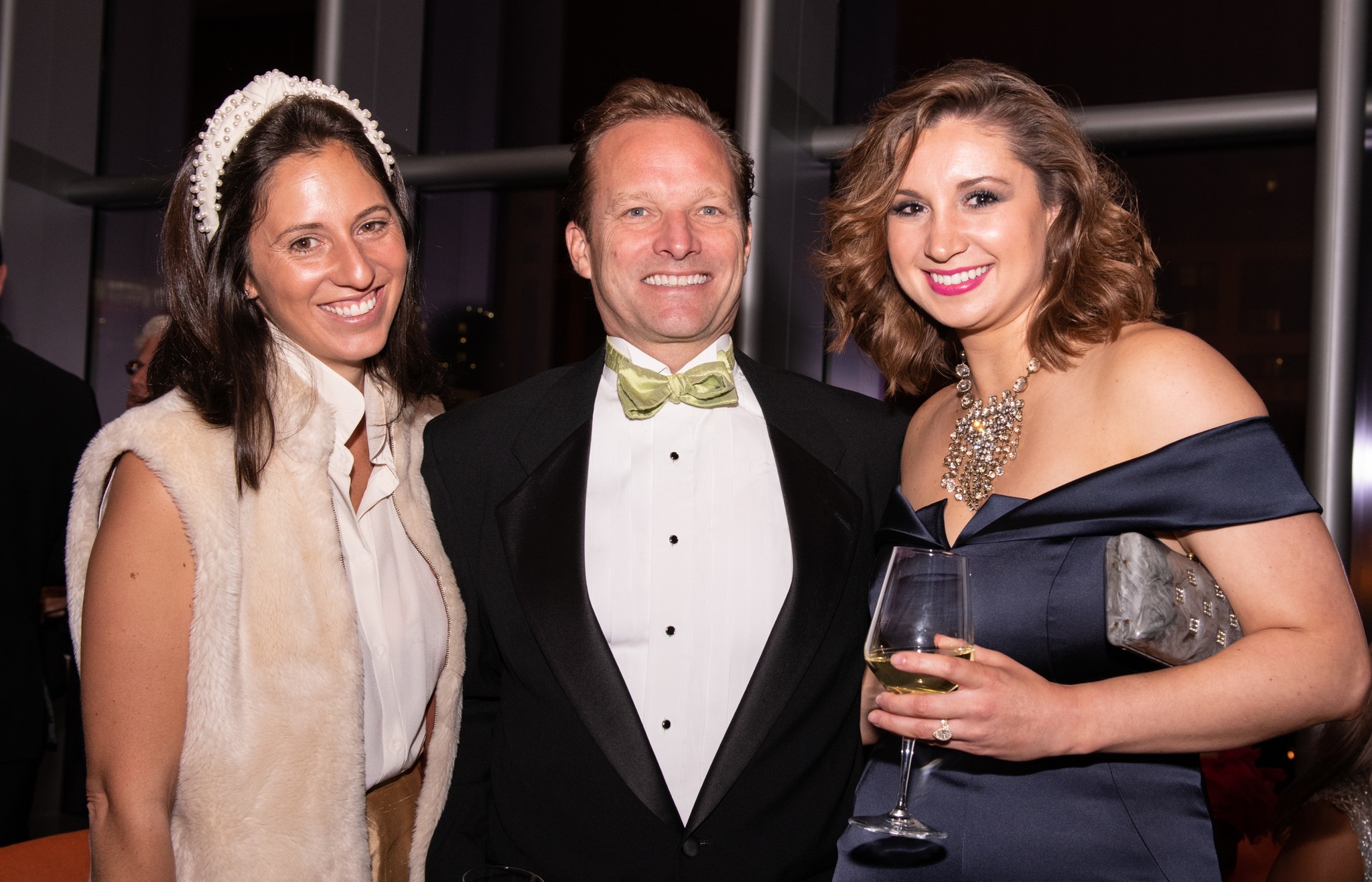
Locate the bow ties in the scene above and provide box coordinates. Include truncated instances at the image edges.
[604,337,738,420]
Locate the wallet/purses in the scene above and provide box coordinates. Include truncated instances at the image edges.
[1103,532,1242,669]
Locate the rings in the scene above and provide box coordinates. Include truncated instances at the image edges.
[932,719,953,741]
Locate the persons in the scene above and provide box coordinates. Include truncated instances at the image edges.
[425,79,913,882]
[125,314,172,409]
[65,69,475,882]
[806,53,1371,881]
[0,241,105,847]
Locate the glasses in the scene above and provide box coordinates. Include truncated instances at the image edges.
[124,360,147,375]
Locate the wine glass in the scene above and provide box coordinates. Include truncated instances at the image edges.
[848,546,976,841]
